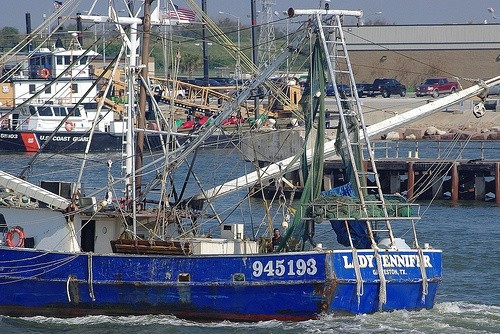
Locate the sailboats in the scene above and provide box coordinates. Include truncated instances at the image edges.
[0,1,500,324]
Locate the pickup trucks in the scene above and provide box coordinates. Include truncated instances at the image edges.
[362,78,407,99]
[415,77,459,99]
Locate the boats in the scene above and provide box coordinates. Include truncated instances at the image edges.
[1,12,197,152]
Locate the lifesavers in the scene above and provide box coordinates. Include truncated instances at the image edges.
[40,68,50,78]
[6,228,25,248]
[65,121,74,131]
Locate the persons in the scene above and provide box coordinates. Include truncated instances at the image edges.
[271,228,287,253]
[325,109,331,129]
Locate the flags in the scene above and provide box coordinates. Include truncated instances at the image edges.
[166,3,195,22]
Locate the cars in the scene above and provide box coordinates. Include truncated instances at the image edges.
[151,76,326,99]
[326,84,352,98]
